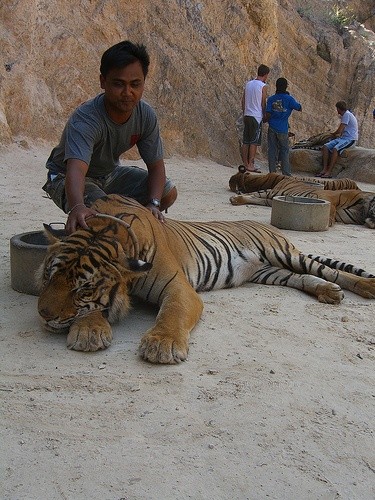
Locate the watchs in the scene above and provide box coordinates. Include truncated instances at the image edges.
[147,198,161,208]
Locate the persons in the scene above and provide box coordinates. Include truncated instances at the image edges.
[264,78,302,176]
[315,101,359,178]
[42,41,177,235]
[241,64,270,173]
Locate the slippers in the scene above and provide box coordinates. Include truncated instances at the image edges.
[247,168,261,173]
[314,171,333,178]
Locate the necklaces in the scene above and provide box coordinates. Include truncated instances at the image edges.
[68,204,85,215]
